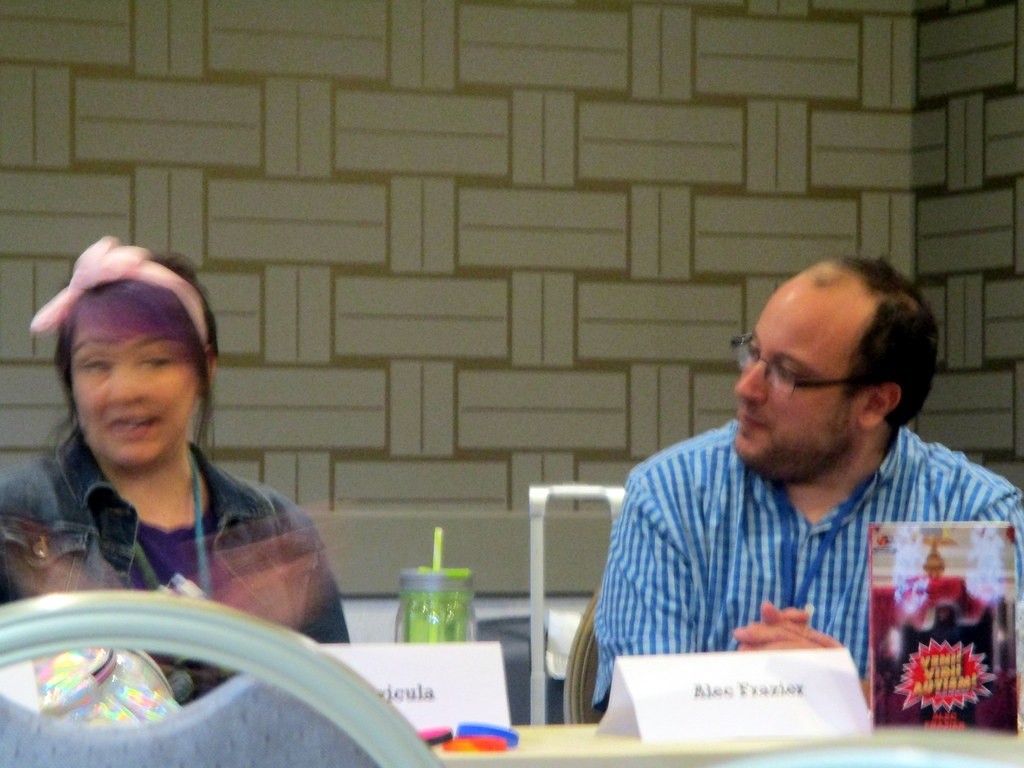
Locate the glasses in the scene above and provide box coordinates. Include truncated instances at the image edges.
[730,334,870,402]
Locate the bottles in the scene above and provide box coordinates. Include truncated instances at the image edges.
[395,569,478,643]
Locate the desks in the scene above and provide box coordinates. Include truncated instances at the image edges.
[432,723,1024,768]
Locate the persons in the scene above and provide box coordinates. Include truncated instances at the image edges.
[0,237,351,706]
[591,254,1024,713]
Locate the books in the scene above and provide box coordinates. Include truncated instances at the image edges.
[868,520,1018,737]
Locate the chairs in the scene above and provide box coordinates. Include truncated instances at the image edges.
[0,591,440,768]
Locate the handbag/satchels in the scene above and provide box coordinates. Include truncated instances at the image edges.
[18,647,178,728]
